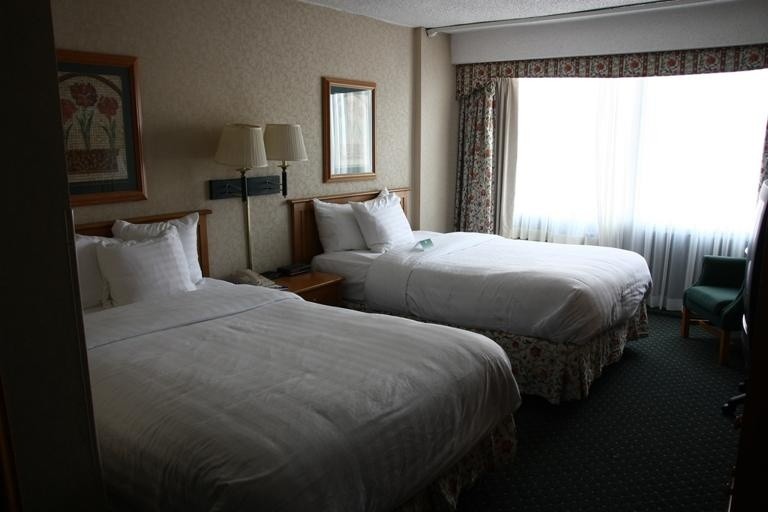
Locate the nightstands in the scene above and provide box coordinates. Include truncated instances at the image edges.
[267,271,346,307]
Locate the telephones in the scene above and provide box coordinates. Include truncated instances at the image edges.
[230,269,276,287]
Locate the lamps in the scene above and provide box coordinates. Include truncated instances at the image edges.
[207,122,309,203]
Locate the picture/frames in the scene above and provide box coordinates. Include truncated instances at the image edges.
[56,48,149,205]
[320,76,378,184]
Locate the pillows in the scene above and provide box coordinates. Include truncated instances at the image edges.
[312,197,368,254]
[112,211,206,285]
[73,234,126,310]
[97,225,197,306]
[348,193,414,254]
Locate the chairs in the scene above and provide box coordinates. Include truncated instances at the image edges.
[677,253,746,363]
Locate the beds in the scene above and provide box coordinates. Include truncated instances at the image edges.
[286,187,654,400]
[73,207,525,509]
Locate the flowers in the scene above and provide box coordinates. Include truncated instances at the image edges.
[57,80,121,152]
